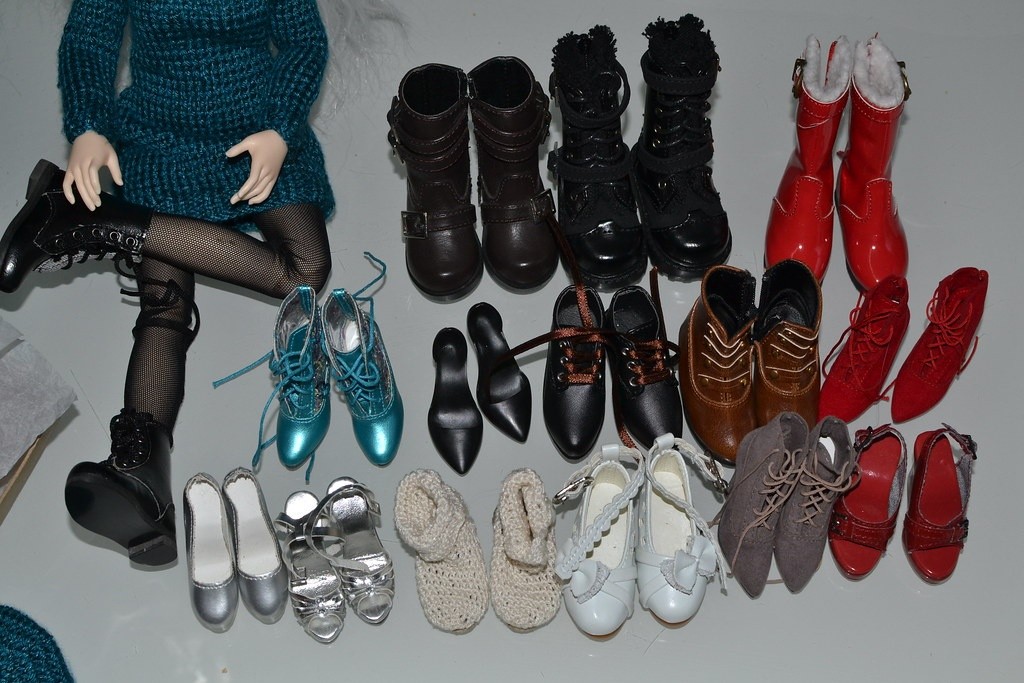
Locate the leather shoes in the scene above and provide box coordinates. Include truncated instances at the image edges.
[431,327,484,475]
[543,283,606,462]
[604,286,684,456]
[467,300,532,442]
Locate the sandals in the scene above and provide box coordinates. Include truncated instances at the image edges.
[307,476,396,624]
[273,489,344,644]
[831,424,909,580]
[903,422,978,584]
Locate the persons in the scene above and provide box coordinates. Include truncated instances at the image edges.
[0,0,415,567]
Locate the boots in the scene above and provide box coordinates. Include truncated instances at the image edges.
[1,158,154,293]
[832,36,908,297]
[468,54,561,295]
[548,24,648,291]
[388,61,484,304]
[64,409,178,565]
[753,258,824,434]
[678,265,760,467]
[321,290,405,465]
[273,283,329,471]
[763,32,854,291]
[628,13,733,283]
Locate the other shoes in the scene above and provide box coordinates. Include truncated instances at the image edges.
[638,432,728,625]
[554,444,636,636]
[183,471,240,635]
[490,467,562,634]
[394,469,490,632]
[220,466,289,624]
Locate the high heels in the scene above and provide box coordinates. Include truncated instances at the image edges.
[773,415,863,594]
[816,273,911,430]
[890,267,989,425]
[716,411,810,598]
